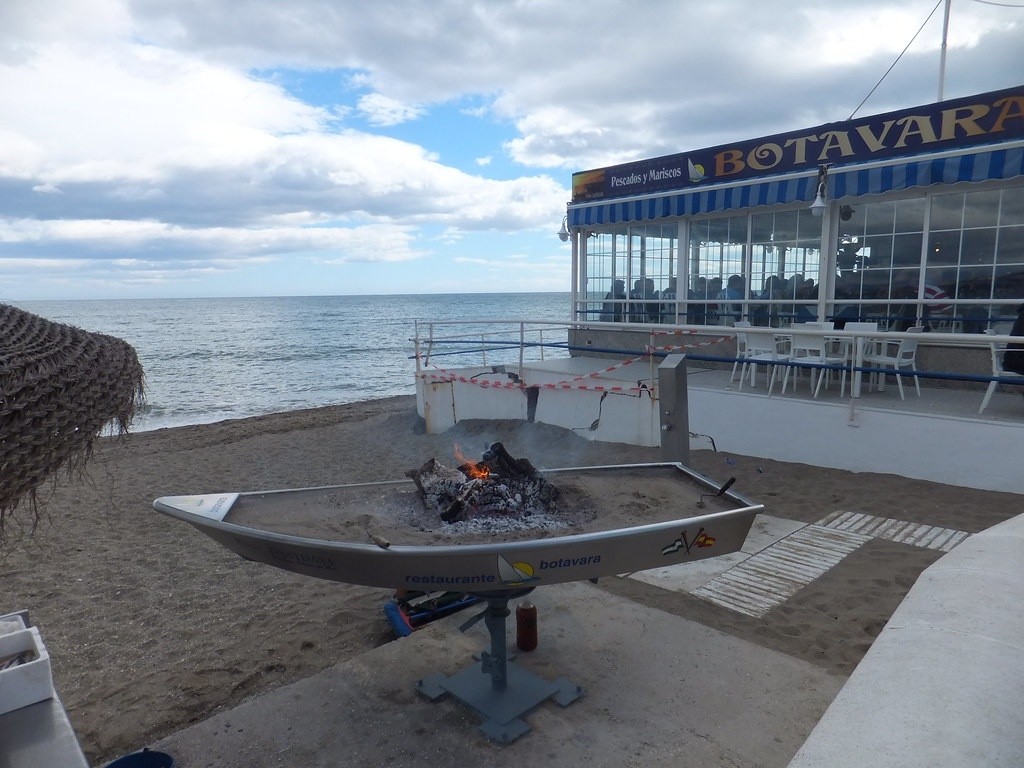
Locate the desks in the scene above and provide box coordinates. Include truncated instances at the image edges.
[751,334,888,397]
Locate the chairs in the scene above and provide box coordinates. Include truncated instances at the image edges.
[799,321,834,385]
[977,328,1024,414]
[864,326,924,400]
[599,293,963,333]
[739,326,797,396]
[781,323,848,399]
[837,322,878,389]
[730,321,779,385]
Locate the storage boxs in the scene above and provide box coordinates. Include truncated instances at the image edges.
[0,615,27,637]
[0,626,53,715]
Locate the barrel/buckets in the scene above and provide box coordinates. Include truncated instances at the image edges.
[103,746,176,768]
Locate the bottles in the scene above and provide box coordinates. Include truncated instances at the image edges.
[516,597,538,652]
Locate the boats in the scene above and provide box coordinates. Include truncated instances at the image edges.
[151,462,766,595]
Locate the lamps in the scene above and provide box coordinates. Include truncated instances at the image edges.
[840,205,856,221]
[809,182,827,216]
[766,245,773,253]
[557,215,567,240]
[561,218,569,242]
[806,248,815,256]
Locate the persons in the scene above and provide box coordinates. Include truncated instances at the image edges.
[1003,303,1024,375]
[834,269,1014,333]
[599,274,819,327]
